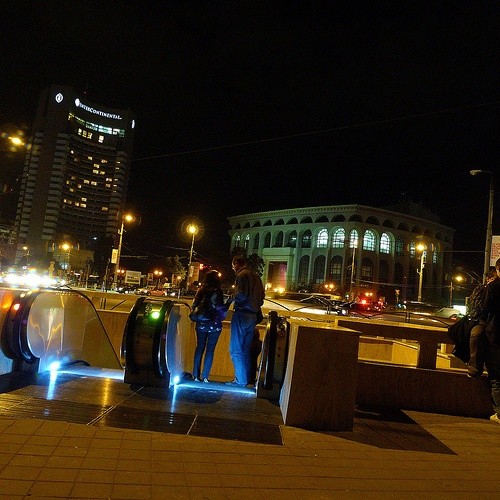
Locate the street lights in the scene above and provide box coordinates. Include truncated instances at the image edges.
[448,276,465,307]
[112,215,132,292]
[418,243,426,300]
[185,225,197,295]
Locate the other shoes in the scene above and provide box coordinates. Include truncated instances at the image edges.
[203,378,209,383]
[225,378,245,388]
[195,377,199,382]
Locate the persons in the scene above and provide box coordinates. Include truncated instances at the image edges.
[73,275,77,286]
[168,288,184,299]
[101,280,106,292]
[467,258,500,423]
[190,269,234,383]
[225,256,266,388]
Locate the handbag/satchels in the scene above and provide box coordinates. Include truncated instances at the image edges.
[189,308,216,322]
[447,313,480,343]
[452,344,470,364]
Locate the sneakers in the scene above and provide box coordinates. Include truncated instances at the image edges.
[490,413,500,424]
[467,372,475,378]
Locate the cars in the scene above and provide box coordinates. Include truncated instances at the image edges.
[135,286,166,295]
[116,285,135,295]
